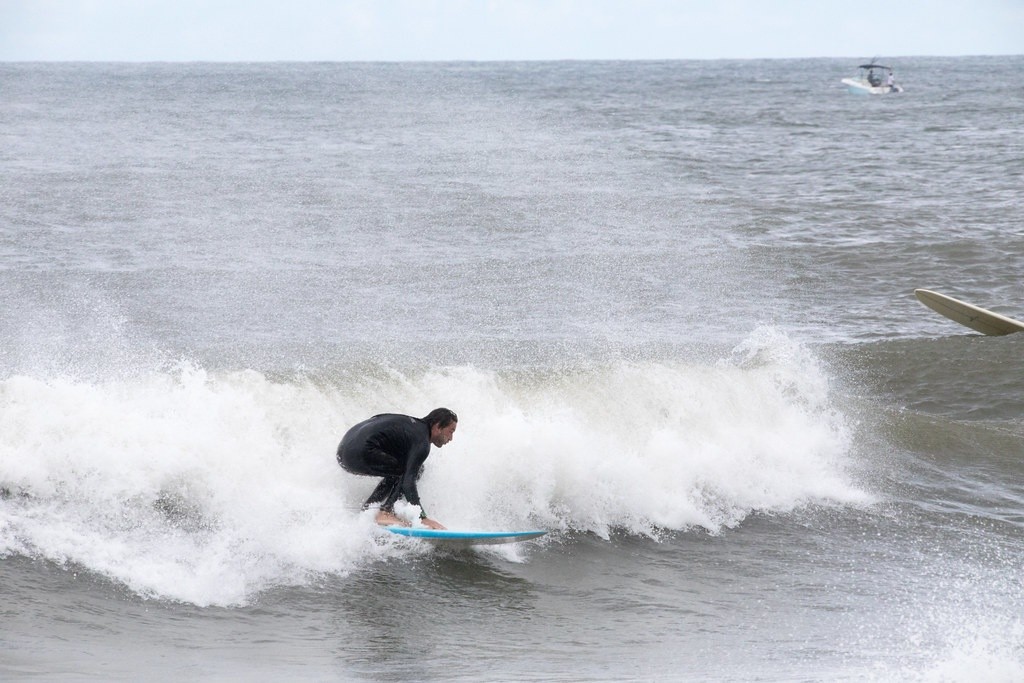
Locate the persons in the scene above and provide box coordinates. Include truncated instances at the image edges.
[867,70,875,87]
[337,408,458,529]
[888,73,894,87]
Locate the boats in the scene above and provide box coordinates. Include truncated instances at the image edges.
[841,76,904,96]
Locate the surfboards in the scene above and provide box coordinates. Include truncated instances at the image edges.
[914,289,1022,334]
[384,527,547,547]
